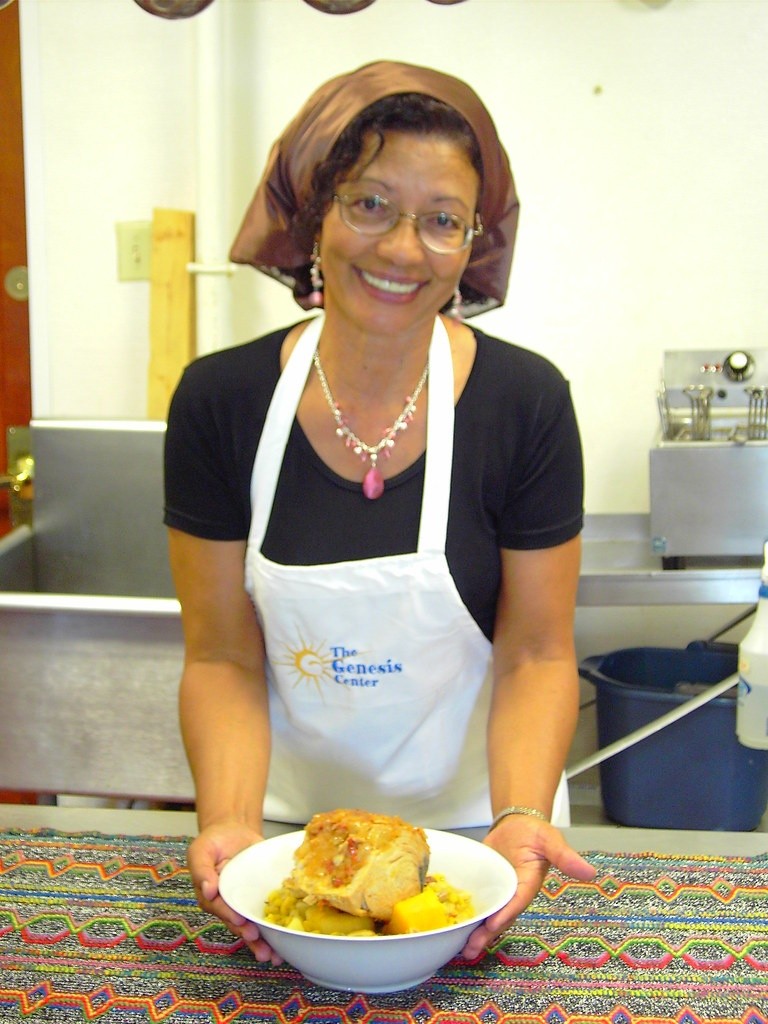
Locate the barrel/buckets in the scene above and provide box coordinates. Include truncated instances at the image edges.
[579,641,768,830]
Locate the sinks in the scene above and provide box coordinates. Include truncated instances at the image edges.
[1,524,200,806]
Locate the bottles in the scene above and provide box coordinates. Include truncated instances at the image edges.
[737,584,768,750]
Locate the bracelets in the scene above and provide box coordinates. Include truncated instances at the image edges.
[488,807,547,831]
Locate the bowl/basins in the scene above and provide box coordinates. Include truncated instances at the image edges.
[218,826,517,993]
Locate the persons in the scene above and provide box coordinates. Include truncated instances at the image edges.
[160,64,601,968]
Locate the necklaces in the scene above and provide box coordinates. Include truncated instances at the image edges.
[313,349,430,501]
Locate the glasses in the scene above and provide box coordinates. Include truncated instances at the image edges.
[329,191,483,254]
